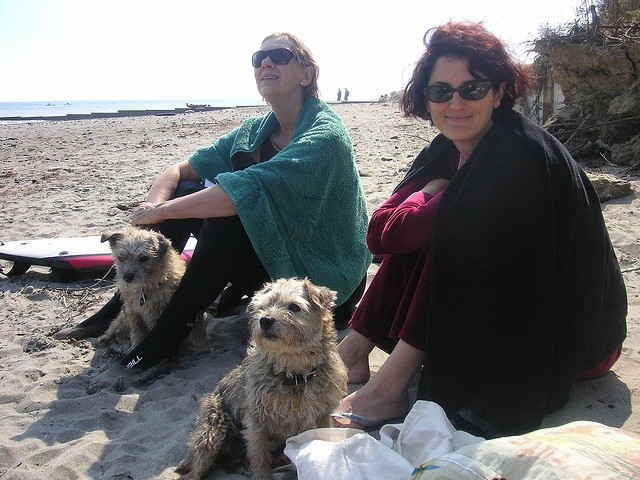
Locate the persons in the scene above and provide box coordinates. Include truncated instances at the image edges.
[333,19,627,433]
[379,95,384,100]
[385,94,389,100]
[54,32,368,392]
[344,88,349,100]
[337,89,341,100]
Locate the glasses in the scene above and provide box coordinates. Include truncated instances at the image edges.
[422,80,494,103]
[252,48,299,67]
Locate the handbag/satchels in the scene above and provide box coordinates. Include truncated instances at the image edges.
[283,400,486,480]
[410,420,640,480]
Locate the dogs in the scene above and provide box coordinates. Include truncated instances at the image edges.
[174,275,351,480]
[98,226,213,356]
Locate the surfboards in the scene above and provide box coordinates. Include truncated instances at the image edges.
[0,236,198,280]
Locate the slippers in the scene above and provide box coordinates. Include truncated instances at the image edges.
[329,410,406,433]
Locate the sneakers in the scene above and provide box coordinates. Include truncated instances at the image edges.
[86,343,179,395]
[53,305,112,340]
